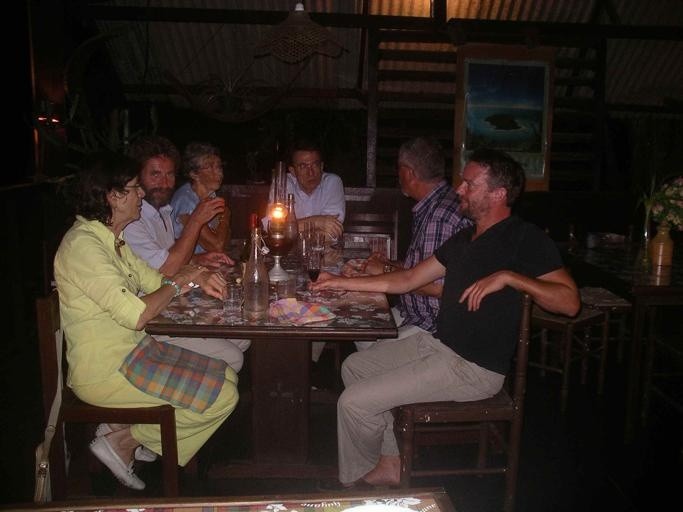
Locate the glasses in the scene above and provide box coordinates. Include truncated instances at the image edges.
[124,182,145,190]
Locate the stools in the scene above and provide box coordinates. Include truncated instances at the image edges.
[532,305,606,414]
[576,286,632,388]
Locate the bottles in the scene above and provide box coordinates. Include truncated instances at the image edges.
[241,213,265,264]
[267,255,290,305]
[286,193,298,247]
[244,228,268,320]
[649,226,672,269]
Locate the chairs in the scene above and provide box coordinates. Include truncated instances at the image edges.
[340,210,400,264]
[399,292,532,512]
[36,290,181,501]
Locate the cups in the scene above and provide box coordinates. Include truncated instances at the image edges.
[296,219,312,257]
[305,250,322,296]
[221,282,243,324]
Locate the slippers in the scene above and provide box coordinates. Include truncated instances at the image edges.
[314,475,392,493]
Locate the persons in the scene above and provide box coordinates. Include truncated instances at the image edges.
[263,143,346,246]
[169,143,233,254]
[55,155,240,490]
[122,134,251,373]
[307,148,580,489]
[354,134,472,351]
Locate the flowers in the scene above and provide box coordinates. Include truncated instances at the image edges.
[643,175,683,229]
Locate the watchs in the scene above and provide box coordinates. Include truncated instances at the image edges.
[383,262,392,274]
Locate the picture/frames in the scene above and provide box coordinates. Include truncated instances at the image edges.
[451,53,556,192]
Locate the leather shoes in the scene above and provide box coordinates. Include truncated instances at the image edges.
[95,423,158,461]
[91,436,147,491]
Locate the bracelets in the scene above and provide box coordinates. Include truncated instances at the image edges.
[159,280,182,296]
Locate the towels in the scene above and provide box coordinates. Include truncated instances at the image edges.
[341,258,370,277]
[268,296,335,325]
[116,335,228,413]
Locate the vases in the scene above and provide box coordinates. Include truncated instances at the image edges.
[652,228,676,285]
